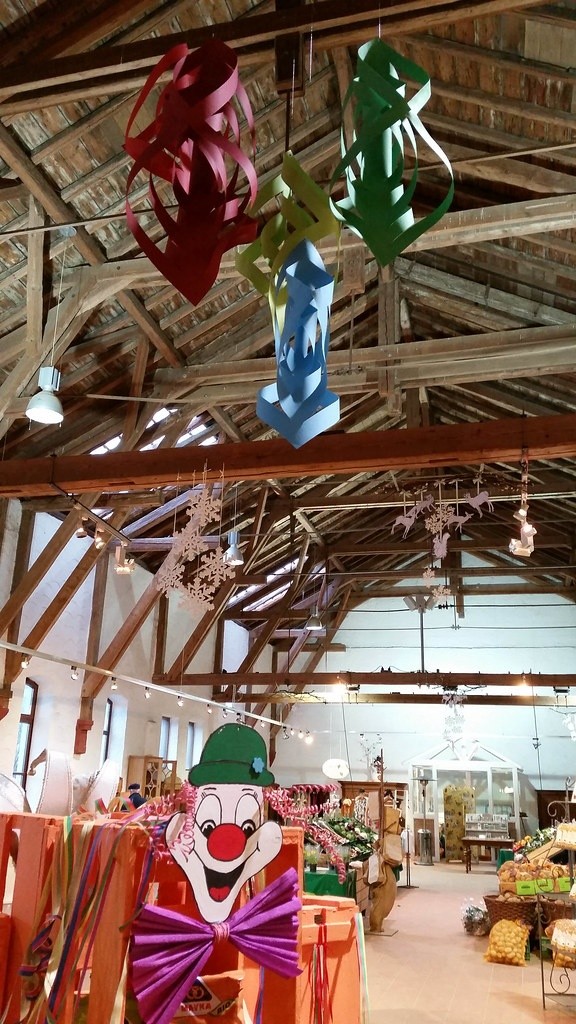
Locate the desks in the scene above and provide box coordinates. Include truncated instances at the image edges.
[461,836,515,873]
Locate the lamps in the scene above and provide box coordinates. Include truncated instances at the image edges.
[222,480,244,566]
[25,225,76,424]
[306,537,323,631]
[509,456,538,557]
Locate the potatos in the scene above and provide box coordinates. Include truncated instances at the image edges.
[483,918,576,971]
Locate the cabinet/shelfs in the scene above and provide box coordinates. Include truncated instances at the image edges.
[267,784,379,919]
[338,780,408,819]
[499,817,576,895]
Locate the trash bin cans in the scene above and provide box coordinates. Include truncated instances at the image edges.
[417,828,433,858]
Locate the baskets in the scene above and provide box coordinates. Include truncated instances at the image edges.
[483,889,536,929]
[542,900,576,928]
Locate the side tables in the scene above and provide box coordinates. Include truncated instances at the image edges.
[126,755,178,801]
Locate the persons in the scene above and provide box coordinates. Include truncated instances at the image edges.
[120,782,150,810]
